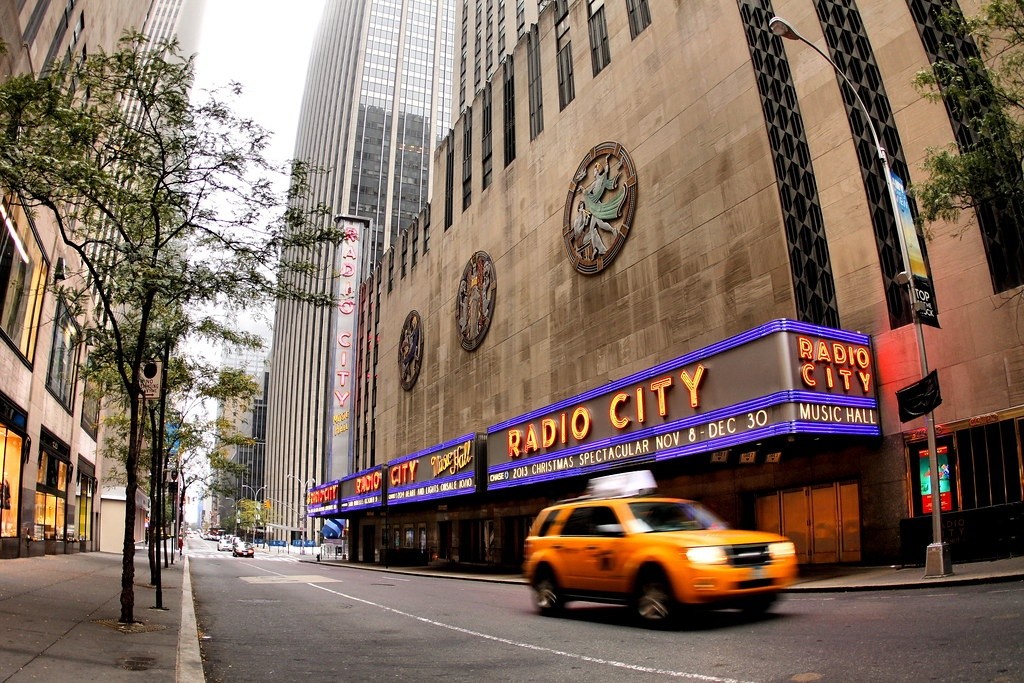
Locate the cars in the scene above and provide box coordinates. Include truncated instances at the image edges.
[233,541,254,559]
[218,539,233,551]
[200,533,243,545]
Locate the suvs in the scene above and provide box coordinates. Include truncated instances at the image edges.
[523,469,801,627]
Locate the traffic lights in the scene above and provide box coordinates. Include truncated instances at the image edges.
[264,500,270,509]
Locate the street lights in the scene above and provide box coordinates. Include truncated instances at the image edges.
[287,474,316,556]
[771,18,948,582]
[242,484,266,544]
[226,498,245,535]
